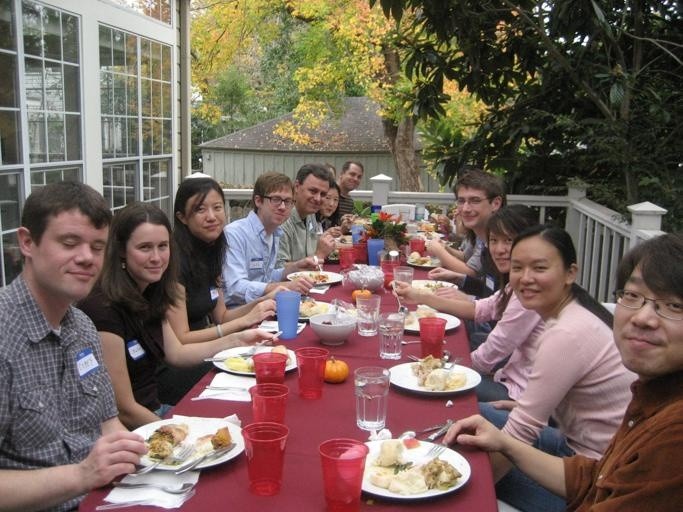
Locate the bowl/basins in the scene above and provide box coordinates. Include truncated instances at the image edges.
[309,313,356,346]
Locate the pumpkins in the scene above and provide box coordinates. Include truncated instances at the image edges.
[352,284,372,303]
[323,355,349,384]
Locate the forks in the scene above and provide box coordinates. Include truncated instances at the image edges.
[199,347,257,362]
[420,438,462,460]
[135,441,192,475]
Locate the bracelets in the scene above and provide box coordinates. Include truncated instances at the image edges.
[216,323,224,338]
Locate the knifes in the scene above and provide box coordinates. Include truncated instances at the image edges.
[173,441,238,475]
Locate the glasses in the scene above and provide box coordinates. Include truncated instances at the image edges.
[454,196,491,206]
[260,196,296,210]
[613,290,682,321]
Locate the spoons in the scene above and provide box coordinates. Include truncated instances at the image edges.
[391,282,409,318]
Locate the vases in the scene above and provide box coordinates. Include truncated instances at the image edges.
[384,239,398,251]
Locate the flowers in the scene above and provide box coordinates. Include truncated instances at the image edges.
[357,210,413,247]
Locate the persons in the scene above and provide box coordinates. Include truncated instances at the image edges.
[475,223,642,511]
[165,174,291,343]
[394,206,549,400]
[83,203,280,427]
[272,160,502,279]
[2,179,150,511]
[442,233,683,510]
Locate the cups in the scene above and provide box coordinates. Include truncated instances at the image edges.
[337,221,447,360]
[246,345,369,511]
[274,290,299,340]
[351,365,392,432]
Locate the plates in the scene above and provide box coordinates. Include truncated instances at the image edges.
[337,437,474,500]
[384,310,459,333]
[286,270,342,285]
[406,259,441,267]
[411,279,458,292]
[131,416,248,474]
[383,360,482,395]
[212,345,298,375]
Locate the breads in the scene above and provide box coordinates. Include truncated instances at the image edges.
[270,344,290,364]
[368,440,428,496]
[423,367,449,391]
[195,432,213,456]
[297,299,321,318]
